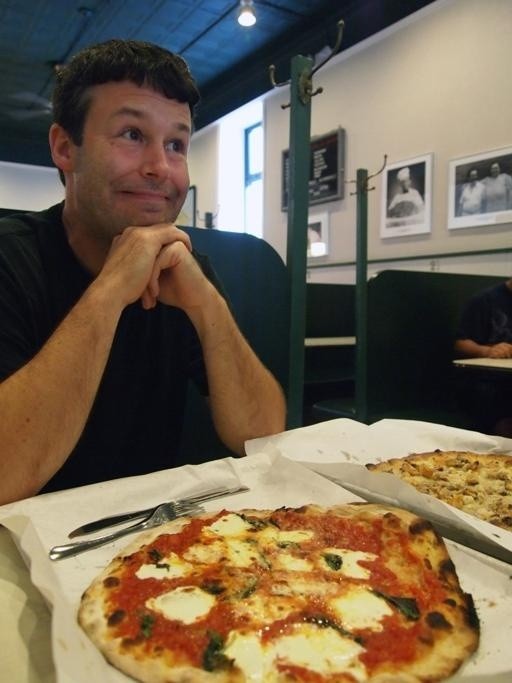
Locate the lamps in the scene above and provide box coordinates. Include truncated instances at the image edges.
[236,1,259,28]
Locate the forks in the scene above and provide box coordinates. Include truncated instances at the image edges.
[51,499,204,558]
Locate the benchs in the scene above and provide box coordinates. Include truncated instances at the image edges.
[170,226,292,428]
[367,268,512,425]
[303,282,369,419]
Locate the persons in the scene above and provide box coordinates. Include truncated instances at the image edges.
[453,276,512,360]
[0,35,289,503]
[384,165,427,218]
[457,159,512,215]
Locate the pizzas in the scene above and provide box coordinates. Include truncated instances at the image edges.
[365,445,511,535]
[75,498,481,682]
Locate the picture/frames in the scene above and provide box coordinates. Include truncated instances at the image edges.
[307,212,331,260]
[447,145,512,231]
[381,154,435,238]
[172,184,198,227]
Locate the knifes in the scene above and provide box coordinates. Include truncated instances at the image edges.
[64,480,248,536]
